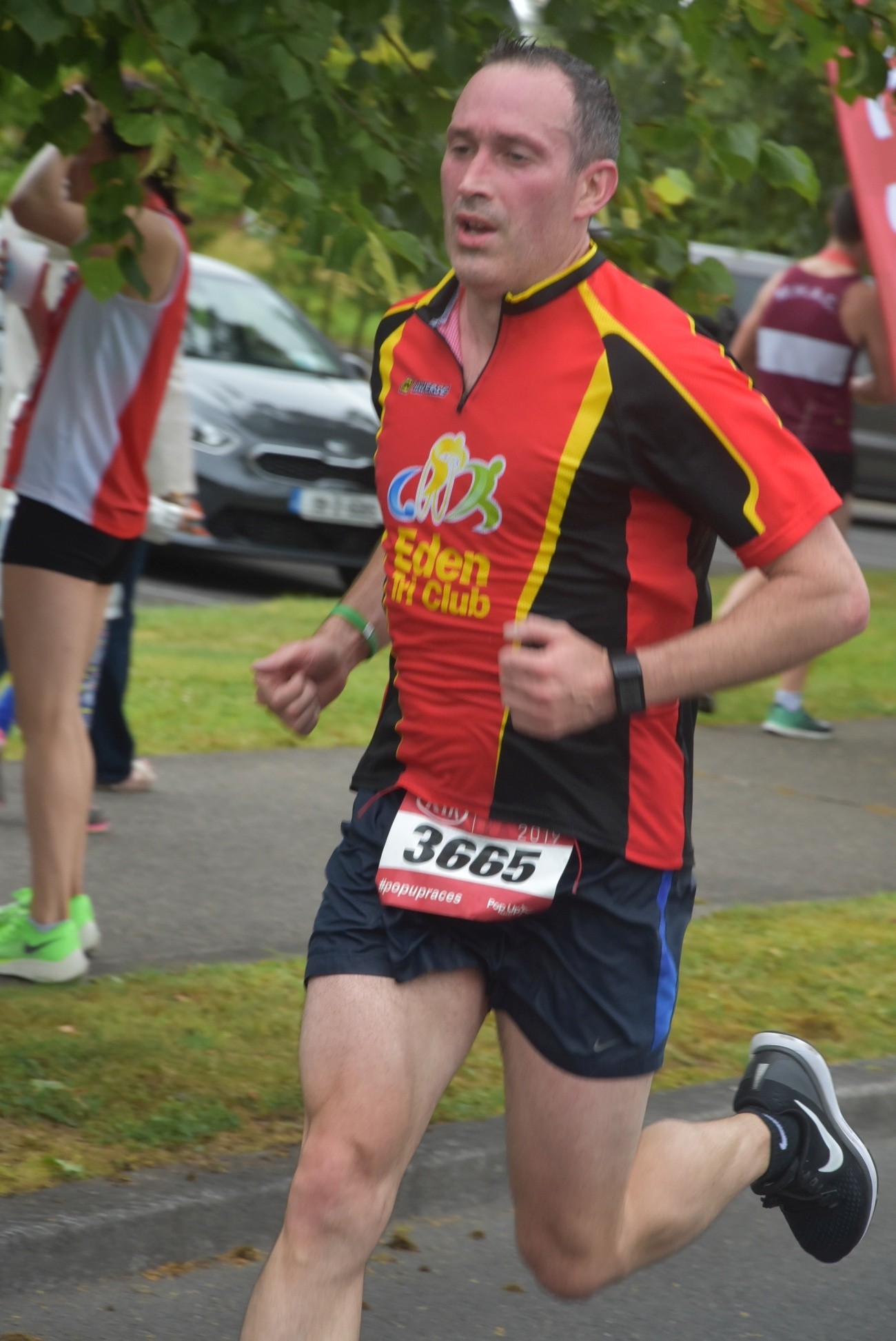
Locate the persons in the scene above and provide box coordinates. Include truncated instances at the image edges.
[700,183,892,739]
[0,64,198,984]
[240,40,874,1341]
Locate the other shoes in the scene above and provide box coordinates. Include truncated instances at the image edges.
[86,805,109,836]
[113,761,153,791]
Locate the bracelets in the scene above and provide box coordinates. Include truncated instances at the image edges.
[328,605,379,659]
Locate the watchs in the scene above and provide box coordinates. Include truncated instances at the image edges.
[608,648,645,715]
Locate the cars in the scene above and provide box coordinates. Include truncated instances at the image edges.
[138,249,396,588]
[587,217,893,508]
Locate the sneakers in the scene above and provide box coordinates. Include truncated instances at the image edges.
[0,921,88,986]
[733,1026,876,1266]
[0,888,102,950]
[760,701,833,743]
